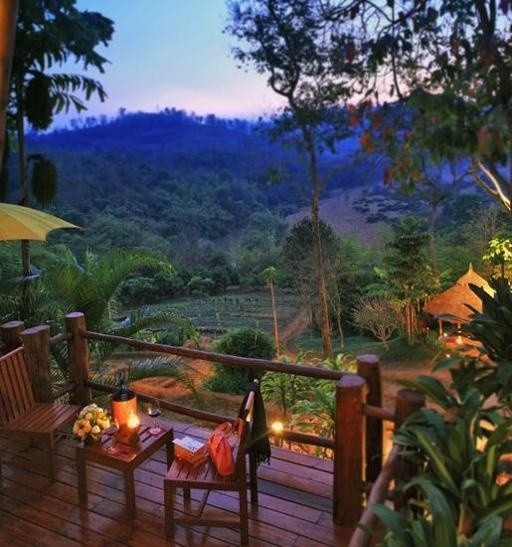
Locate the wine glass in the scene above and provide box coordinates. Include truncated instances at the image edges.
[148,399,161,434]
[106,423,119,453]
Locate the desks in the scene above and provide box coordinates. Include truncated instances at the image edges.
[76,416,174,520]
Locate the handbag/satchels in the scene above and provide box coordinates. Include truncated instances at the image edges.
[207,418,243,477]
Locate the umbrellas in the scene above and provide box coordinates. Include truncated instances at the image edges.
[0,202,83,242]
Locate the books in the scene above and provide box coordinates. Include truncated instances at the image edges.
[171,436,208,468]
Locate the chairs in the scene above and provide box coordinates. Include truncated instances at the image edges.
[163,379,258,547]
[0,347,81,485]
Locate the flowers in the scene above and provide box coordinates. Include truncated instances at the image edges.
[72,403,110,441]
